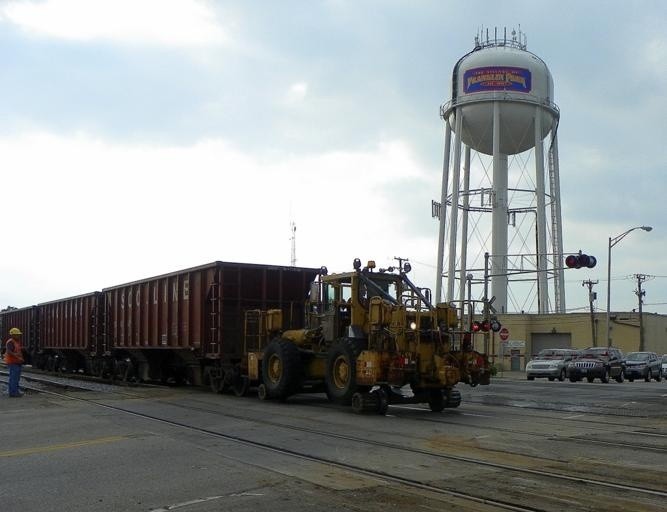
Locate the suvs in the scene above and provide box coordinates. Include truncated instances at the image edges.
[523,342,667,385]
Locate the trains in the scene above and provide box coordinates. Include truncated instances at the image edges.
[1,255,504,415]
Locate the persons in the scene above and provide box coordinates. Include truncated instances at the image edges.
[3,326,25,397]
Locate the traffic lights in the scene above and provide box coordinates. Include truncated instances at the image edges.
[565,253,581,270]
[469,319,501,331]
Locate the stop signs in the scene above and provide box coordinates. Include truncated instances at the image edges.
[499,327,511,341]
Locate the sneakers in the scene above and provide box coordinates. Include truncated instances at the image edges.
[9,392,24,397]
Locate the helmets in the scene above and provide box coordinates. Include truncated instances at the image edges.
[359,284,368,291]
[9,328,23,335]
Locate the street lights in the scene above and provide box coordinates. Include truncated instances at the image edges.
[605,225,654,347]
